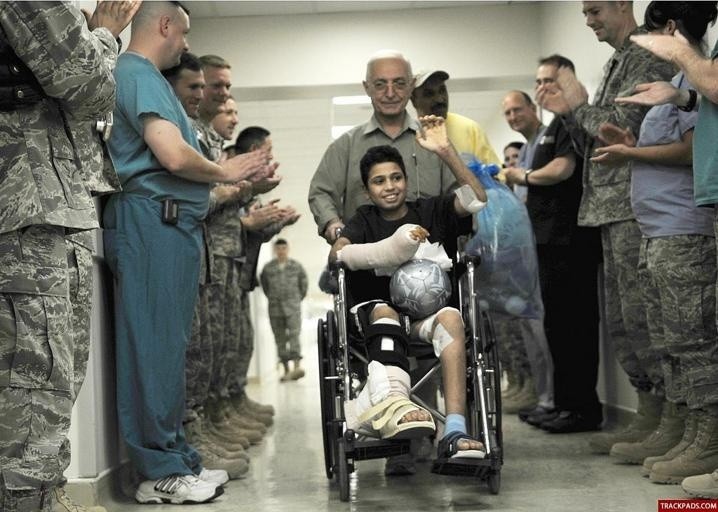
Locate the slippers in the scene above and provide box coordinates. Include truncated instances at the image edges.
[437,431,487,459]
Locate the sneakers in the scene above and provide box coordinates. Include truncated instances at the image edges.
[410,436,433,462]
[194,467,230,485]
[134,474,224,504]
[384,453,416,475]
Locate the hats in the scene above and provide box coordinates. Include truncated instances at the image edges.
[412,70,450,88]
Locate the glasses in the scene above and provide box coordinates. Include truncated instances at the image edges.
[374,80,409,91]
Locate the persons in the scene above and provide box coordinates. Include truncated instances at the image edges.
[0,1,140,512]
[497,0,718,504]
[308,52,465,475]
[329,116,488,464]
[410,70,501,177]
[103,0,308,507]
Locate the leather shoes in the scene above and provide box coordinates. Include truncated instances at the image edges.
[519,406,603,433]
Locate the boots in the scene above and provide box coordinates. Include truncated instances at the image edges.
[292,360,304,382]
[52,488,106,512]
[279,363,290,381]
[590,392,718,499]
[501,370,539,414]
[184,392,274,479]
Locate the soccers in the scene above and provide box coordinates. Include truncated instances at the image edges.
[390,259,452,319]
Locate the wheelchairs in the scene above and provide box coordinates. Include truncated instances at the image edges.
[316,228,504,503]
